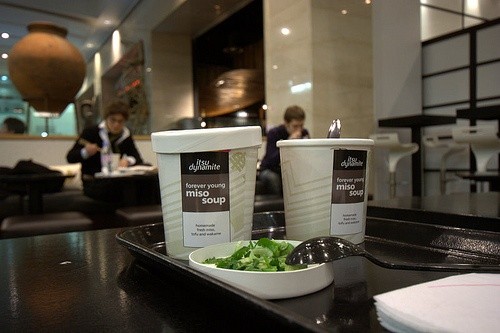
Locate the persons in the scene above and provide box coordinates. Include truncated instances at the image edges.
[257,105,311,196]
[2,118,27,133]
[66,102,145,178]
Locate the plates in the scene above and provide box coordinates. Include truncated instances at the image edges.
[187,239,334,299]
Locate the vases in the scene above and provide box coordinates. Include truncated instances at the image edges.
[7,22,86,117]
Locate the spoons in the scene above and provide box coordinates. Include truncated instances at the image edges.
[283,235,500,274]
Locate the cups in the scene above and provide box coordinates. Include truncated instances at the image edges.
[102,153,120,176]
[275,138,376,244]
[149,126,262,261]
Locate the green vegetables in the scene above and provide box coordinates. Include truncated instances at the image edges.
[202,238,308,273]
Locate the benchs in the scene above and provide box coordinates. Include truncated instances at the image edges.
[0,189,98,214]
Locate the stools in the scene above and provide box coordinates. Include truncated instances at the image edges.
[116,205,163,227]
[371,122,500,200]
[0,212,93,239]
[254,195,284,212]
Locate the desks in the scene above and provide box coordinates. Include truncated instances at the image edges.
[0,174,74,213]
[84,167,157,181]
[456,104,500,122]
[0,193,500,333]
[379,115,456,197]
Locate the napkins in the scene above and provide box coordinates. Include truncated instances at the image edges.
[373,273,500,333]
